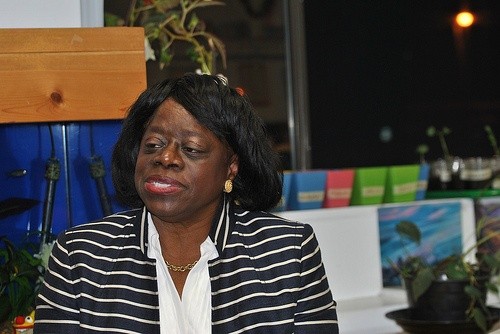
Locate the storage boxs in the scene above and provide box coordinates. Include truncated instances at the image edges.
[291,169,327,209]
[416,165,430,199]
[384,166,419,202]
[353,167,387,204]
[321,170,355,207]
[274,171,292,210]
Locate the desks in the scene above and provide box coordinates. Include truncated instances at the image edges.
[278,198,475,293]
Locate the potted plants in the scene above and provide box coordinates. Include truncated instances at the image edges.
[386,218,500,334]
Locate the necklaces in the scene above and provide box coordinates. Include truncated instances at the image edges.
[164,259,199,273]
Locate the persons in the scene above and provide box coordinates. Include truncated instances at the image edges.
[31,71,340,334]
